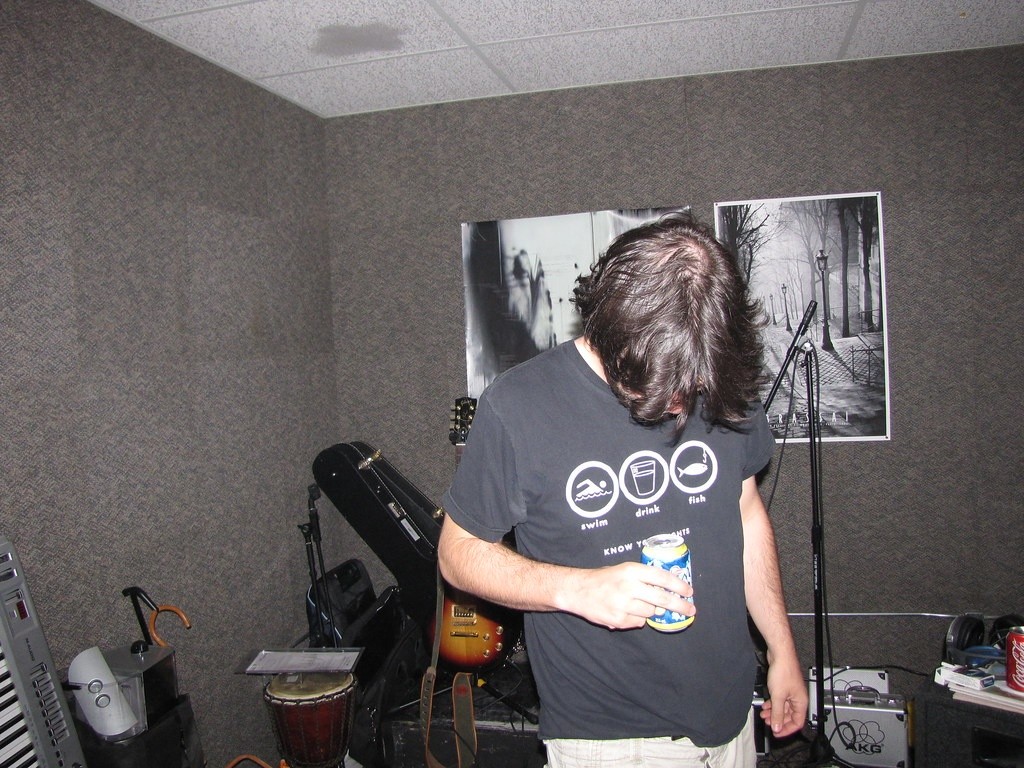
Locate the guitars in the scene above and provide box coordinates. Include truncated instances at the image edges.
[424,396,525,682]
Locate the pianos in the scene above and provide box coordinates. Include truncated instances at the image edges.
[0,539,89,768]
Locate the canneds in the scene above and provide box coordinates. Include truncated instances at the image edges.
[640,533,694,633]
[1005,626,1024,692]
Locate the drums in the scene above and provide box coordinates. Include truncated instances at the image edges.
[261,670,359,768]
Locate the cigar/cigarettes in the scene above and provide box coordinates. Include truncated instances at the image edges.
[941,662,954,668]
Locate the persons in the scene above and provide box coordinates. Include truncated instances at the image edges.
[437,216,809,767]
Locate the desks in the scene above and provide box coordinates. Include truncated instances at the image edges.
[914,676,1023,768]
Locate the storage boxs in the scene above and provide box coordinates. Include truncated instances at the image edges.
[57,643,176,745]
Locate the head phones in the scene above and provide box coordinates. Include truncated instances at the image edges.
[943,614,1024,666]
